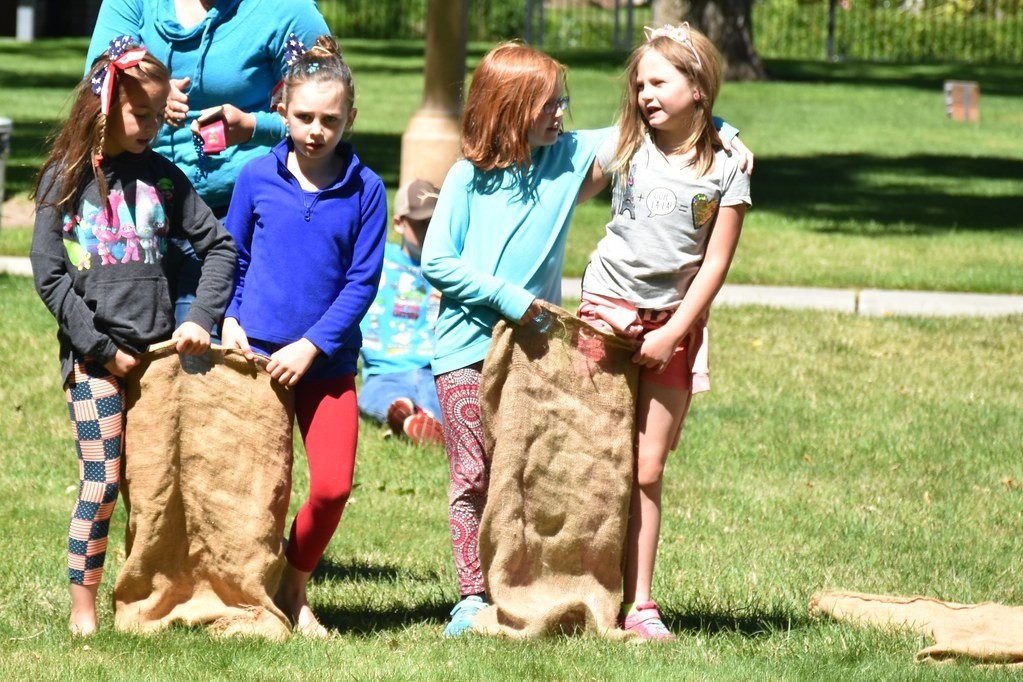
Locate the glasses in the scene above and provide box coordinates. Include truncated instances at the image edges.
[543,96,570,114]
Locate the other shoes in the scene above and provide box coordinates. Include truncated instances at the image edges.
[387,397,415,434]
[403,412,447,446]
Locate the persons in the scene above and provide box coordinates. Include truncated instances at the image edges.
[85,0,336,340]
[574,24,751,644]
[216,32,388,641]
[421,39,753,635]
[29,34,241,636]
[357,180,445,450]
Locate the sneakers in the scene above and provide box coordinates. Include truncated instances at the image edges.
[442,593,491,637]
[617,603,677,643]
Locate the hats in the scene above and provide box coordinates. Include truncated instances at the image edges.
[394,179,441,220]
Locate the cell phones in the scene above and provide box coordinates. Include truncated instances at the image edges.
[196,106,224,127]
